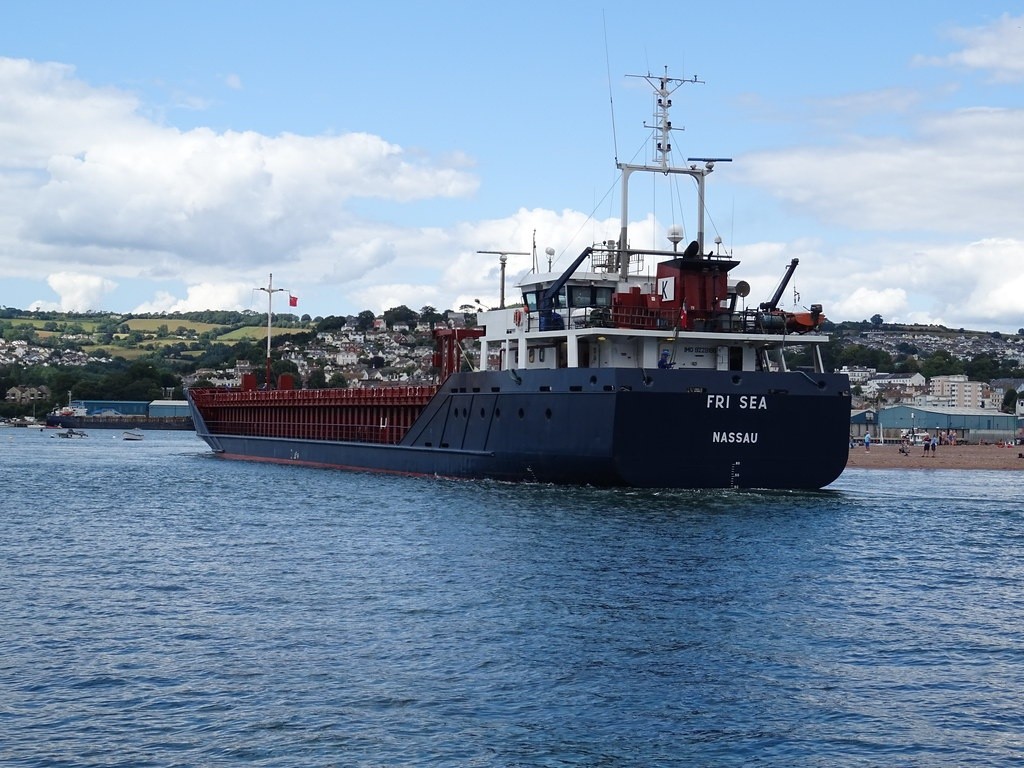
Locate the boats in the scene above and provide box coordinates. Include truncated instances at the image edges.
[122,430,144,441]
[52,427,89,440]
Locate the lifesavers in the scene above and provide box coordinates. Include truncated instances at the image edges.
[513,309,522,328]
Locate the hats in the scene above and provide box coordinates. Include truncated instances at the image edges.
[663,351,669,356]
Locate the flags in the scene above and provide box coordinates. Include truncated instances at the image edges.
[681,304,688,330]
[290,296,298,307]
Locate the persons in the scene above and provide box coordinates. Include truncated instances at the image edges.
[941,430,948,445]
[906,431,911,437]
[948,429,954,446]
[921,433,930,457]
[997,438,1004,448]
[901,439,911,456]
[659,351,675,371]
[914,428,920,436]
[931,434,937,458]
[938,429,943,446]
[863,429,872,454]
[875,429,879,444]
[900,431,904,436]
[850,431,855,449]
[951,430,958,446]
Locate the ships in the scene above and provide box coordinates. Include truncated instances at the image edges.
[181,62,855,498]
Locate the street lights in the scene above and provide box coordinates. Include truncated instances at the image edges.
[911,413,915,432]
